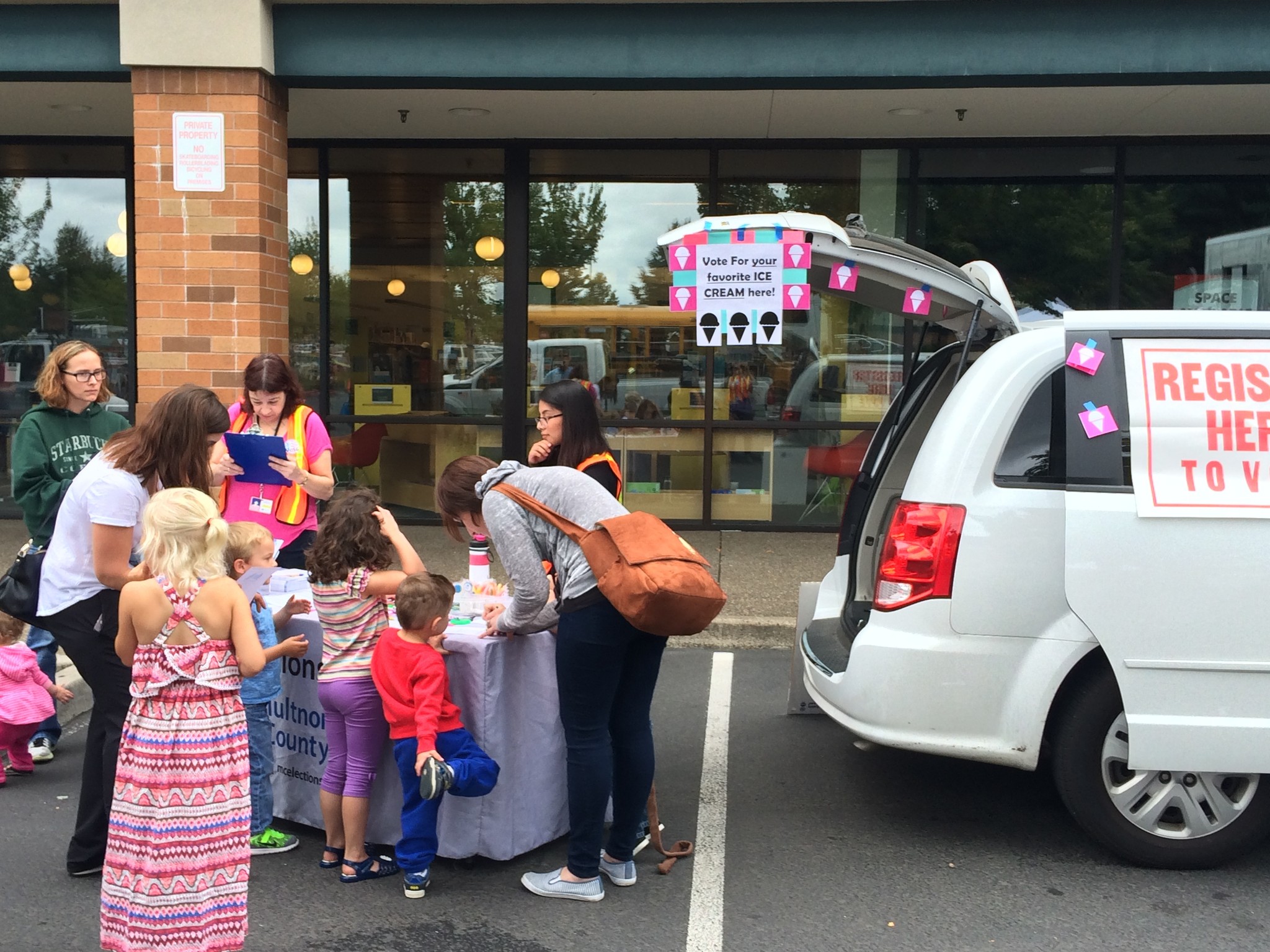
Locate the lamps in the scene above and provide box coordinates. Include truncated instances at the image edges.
[387,265,405,296]
[540,268,561,289]
[107,209,127,258]
[472,233,506,263]
[9,260,35,291]
[290,252,314,275]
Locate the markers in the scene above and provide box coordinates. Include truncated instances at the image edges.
[388,606,396,620]
[496,605,499,609]
[473,583,508,596]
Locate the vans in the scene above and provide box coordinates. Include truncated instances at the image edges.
[443,343,502,373]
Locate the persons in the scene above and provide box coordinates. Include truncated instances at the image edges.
[0,340,667,952]
[447,347,760,422]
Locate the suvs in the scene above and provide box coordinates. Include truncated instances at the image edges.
[757,325,936,448]
[656,209,1270,875]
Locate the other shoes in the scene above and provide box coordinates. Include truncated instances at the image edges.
[643,822,664,839]
[521,867,604,901]
[598,849,638,886]
[633,838,649,855]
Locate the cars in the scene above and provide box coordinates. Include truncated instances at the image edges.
[291,343,321,354]
[0,340,131,421]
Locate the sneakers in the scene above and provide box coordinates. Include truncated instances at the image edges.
[419,757,453,801]
[403,877,430,899]
[27,738,55,761]
[4,764,34,776]
[250,827,299,855]
[67,855,105,876]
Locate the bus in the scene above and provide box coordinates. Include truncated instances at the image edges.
[524,302,798,390]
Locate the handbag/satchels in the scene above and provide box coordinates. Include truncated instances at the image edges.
[579,511,727,636]
[0,545,51,631]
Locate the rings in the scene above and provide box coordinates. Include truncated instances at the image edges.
[227,467,230,471]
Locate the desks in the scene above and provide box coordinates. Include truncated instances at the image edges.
[323,412,780,526]
[262,584,613,862]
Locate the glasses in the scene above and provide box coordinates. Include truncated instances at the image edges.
[534,414,562,428]
[60,368,106,382]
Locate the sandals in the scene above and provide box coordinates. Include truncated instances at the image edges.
[341,857,400,882]
[320,846,346,868]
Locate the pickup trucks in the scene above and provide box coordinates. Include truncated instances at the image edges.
[443,337,772,418]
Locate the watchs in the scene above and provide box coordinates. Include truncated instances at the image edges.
[297,470,309,486]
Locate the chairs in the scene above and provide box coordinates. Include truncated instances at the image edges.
[796,430,876,523]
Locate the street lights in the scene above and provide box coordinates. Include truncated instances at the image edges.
[38,306,44,332]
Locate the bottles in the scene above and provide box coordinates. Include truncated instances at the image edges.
[468,534,494,583]
[454,578,497,594]
